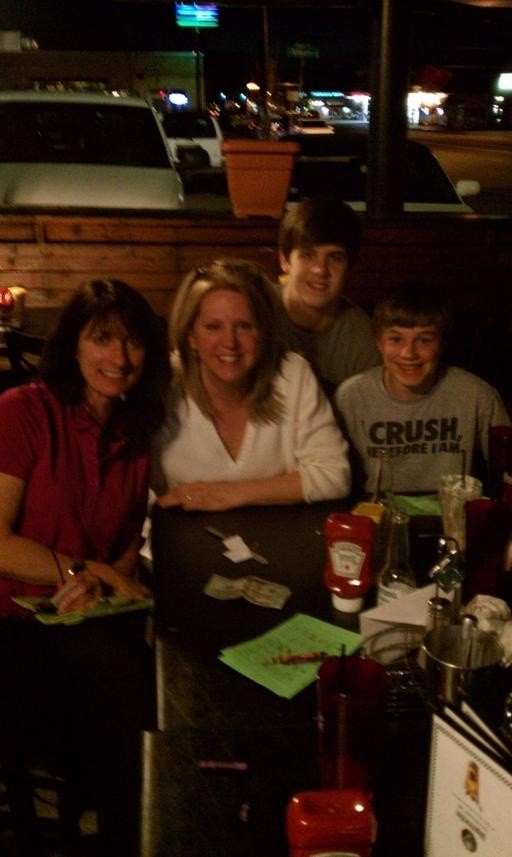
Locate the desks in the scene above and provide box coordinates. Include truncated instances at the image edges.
[143,497,512,857]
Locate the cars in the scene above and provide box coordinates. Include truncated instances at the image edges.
[291,118,335,135]
[1,91,184,214]
[280,132,484,217]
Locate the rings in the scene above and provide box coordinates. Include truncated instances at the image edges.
[186,491,192,502]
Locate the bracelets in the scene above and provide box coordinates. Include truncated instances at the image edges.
[64,556,87,575]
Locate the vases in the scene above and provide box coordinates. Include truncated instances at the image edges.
[220,141,303,221]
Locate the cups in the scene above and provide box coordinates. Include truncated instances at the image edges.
[440,475,483,548]
[318,656,386,797]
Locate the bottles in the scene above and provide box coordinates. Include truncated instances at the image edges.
[377,514,417,602]
[425,561,484,672]
[372,445,397,514]
[323,512,375,614]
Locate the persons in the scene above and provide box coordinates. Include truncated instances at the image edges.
[328,290,512,492]
[0,276,168,624]
[260,197,385,400]
[150,255,354,515]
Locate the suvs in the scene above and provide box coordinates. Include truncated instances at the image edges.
[161,106,223,171]
